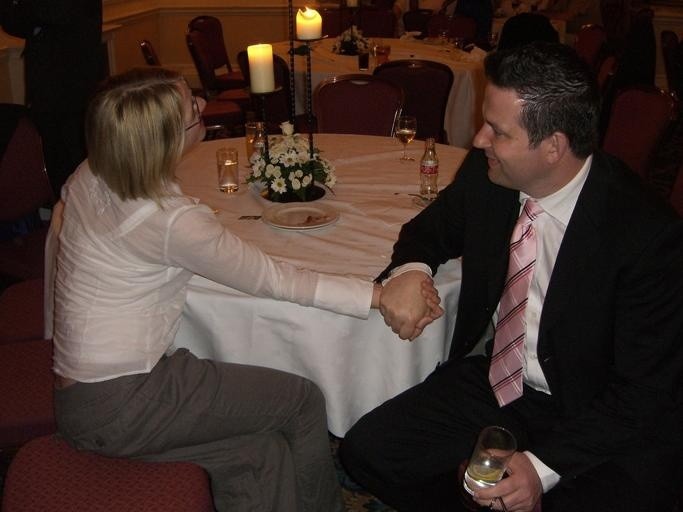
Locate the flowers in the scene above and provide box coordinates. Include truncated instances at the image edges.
[331,24,370,54]
[242,120,337,200]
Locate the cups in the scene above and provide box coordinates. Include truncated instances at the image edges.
[246,123,264,162]
[217,147,238,193]
[464,426,517,497]
[358,49,369,70]
[450,37,463,59]
[373,44,390,65]
[438,30,449,55]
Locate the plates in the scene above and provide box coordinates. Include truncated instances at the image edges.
[262,202,338,231]
[413,195,437,210]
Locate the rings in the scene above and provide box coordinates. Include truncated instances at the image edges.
[498,496,507,511]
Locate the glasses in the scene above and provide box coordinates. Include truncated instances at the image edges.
[183,85,202,133]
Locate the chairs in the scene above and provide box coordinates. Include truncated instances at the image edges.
[319,7,466,37]
[0,112,55,278]
[186,32,261,123]
[140,40,244,140]
[312,73,404,137]
[661,30,683,99]
[238,51,318,134]
[573,23,619,83]
[373,59,454,145]
[189,16,245,89]
[601,85,682,213]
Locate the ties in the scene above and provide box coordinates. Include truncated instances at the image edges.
[488,197,543,409]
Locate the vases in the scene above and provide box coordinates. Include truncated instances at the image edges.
[251,179,328,209]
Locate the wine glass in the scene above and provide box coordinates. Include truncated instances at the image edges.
[395,116,417,161]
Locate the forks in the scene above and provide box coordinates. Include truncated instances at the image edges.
[393,192,436,202]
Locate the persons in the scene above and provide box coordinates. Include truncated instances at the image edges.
[43,66,446,512]
[336,36,682,512]
[0,0,105,203]
[338,0,663,95]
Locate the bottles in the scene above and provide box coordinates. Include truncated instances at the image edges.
[421,138,439,199]
[254,122,264,157]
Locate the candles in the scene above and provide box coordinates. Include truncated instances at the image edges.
[247,42,275,92]
[296,5,323,40]
